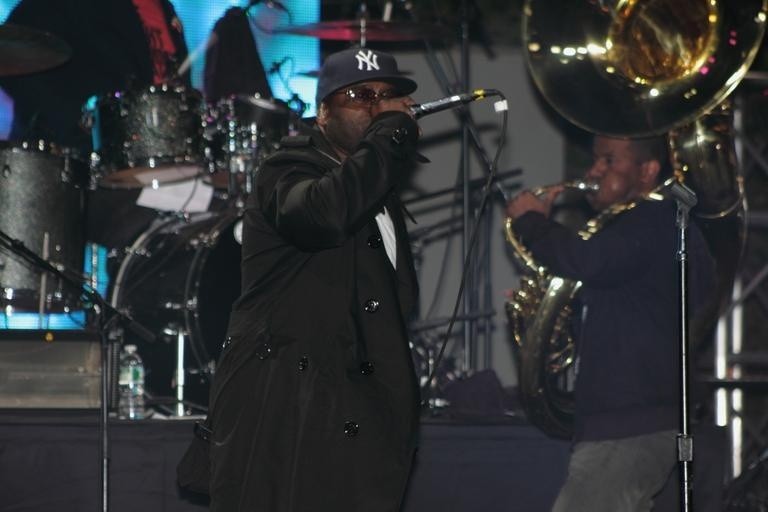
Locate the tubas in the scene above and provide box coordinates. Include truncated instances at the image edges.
[503,0,766,439]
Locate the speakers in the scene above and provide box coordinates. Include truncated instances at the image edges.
[0,329,104,413]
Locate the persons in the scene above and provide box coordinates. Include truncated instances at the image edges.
[172,45,430,512]
[5,0,241,411]
[502,133,723,510]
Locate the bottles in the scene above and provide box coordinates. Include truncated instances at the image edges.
[119,341,144,418]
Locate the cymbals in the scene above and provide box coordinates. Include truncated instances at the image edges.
[271,21,448,42]
[0,25,73,75]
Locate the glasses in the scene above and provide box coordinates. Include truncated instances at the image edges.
[335,86,399,108]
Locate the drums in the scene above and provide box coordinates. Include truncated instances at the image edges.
[207,94,296,194]
[85,87,207,192]
[1,141,88,314]
[110,212,246,414]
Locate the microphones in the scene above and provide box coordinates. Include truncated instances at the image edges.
[658,167,699,209]
[408,91,473,120]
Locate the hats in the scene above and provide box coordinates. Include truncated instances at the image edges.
[316,47,416,107]
